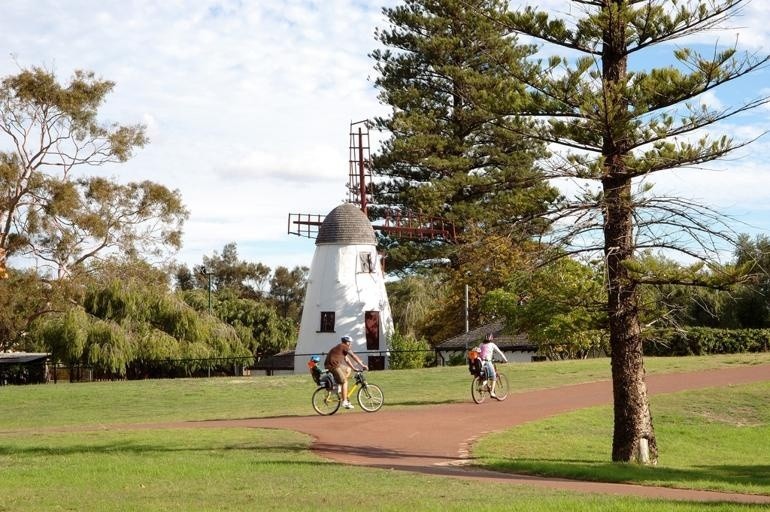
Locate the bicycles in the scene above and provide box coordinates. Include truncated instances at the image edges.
[471,355,510,404]
[311,365,384,416]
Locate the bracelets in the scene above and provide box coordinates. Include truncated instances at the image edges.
[358,361,363,366]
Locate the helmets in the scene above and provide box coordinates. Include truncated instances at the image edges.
[472,347,481,353]
[341,336,352,342]
[485,333,493,341]
[311,356,320,362]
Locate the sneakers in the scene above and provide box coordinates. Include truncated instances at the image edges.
[491,393,496,397]
[341,401,354,409]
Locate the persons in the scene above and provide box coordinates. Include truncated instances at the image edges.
[324,336,369,409]
[310,356,338,391]
[471,347,490,380]
[478,332,508,398]
